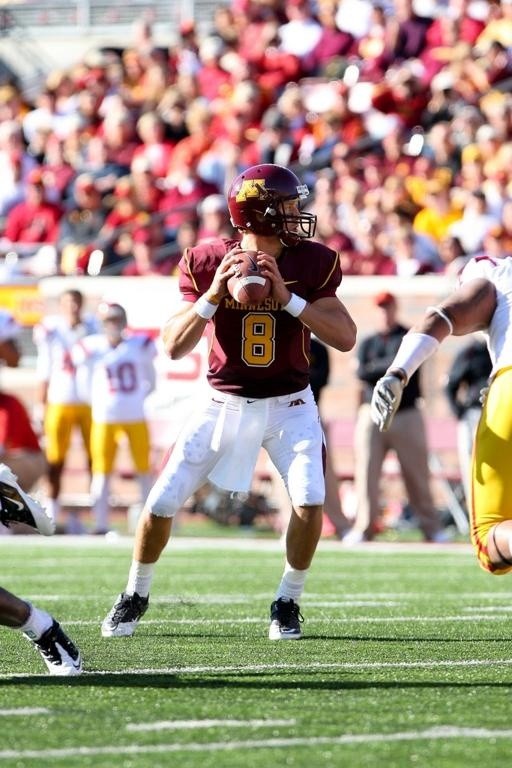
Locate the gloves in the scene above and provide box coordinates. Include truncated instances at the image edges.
[370,375,402,432]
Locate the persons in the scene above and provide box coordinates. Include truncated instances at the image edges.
[445,332,493,493]
[369,256,512,576]
[305,338,352,541]
[1,288,160,538]
[100,165,358,641]
[0,0,511,275]
[341,293,451,546]
[0,392,82,676]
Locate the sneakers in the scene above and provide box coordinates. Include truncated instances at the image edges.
[32,619,82,675]
[101,592,149,637]
[0,462,55,537]
[269,596,302,640]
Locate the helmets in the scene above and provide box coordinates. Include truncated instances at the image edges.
[228,164,309,246]
[99,304,127,332]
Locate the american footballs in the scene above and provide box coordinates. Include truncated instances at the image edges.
[226,250,270,305]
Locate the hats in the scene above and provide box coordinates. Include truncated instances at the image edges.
[375,294,393,306]
